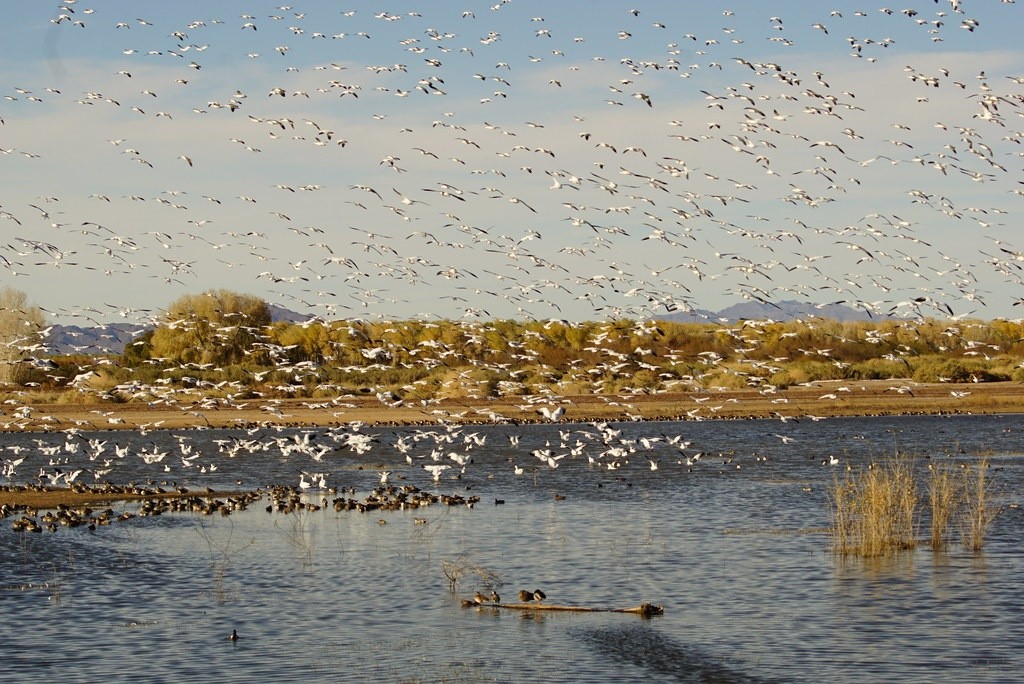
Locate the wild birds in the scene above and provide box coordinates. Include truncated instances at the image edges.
[2,1,1017,608]
